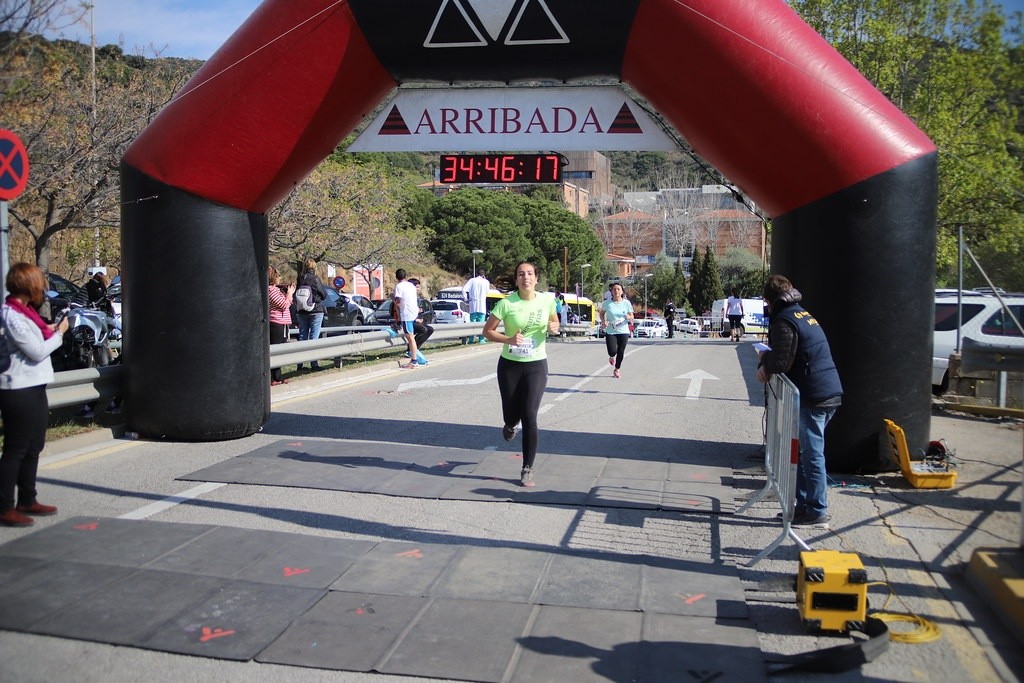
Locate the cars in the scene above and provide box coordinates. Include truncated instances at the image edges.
[430,300,471,324]
[49,273,80,317]
[339,294,377,320]
[105,283,123,331]
[599,320,634,338]
[634,320,667,338]
[634,311,659,320]
[366,299,437,326]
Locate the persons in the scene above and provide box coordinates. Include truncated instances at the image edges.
[77,272,114,318]
[390,269,418,369]
[757,275,843,529]
[0,262,69,527]
[462,269,490,345]
[555,291,566,337]
[393,278,434,364]
[600,284,627,312]
[664,300,675,338]
[726,289,744,342]
[482,261,559,486]
[269,266,296,386]
[600,283,633,378]
[297,259,327,372]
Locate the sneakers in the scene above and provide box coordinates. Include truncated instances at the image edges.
[401,360,419,369]
[72,407,95,418]
[391,324,403,330]
[776,504,807,521]
[520,465,535,487]
[103,405,121,414]
[790,510,829,530]
[502,423,519,441]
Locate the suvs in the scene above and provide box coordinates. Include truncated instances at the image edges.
[672,319,702,334]
[276,286,363,337]
[932,288,1024,392]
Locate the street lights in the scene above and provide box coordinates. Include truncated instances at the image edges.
[645,274,653,318]
[472,249,483,278]
[581,264,591,297]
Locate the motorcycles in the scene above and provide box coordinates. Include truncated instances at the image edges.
[56,275,121,367]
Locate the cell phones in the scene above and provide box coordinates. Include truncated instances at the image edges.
[281,286,288,294]
[57,311,70,326]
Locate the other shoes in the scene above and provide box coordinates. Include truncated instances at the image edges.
[16,501,58,516]
[311,366,325,372]
[0,509,34,528]
[467,340,474,344]
[609,357,616,365]
[297,366,311,374]
[735,335,739,342]
[613,369,621,378]
[404,351,411,358]
[479,339,486,344]
[730,339,734,342]
[271,378,289,386]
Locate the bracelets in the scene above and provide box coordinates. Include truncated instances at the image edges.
[55,330,63,336]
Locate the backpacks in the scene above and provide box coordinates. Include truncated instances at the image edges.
[0,307,21,374]
[295,274,318,314]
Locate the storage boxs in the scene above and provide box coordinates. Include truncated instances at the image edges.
[884,417,957,489]
[799,548,869,634]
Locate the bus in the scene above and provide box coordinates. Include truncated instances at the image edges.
[438,288,595,327]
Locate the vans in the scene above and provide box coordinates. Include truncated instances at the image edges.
[711,297,769,337]
